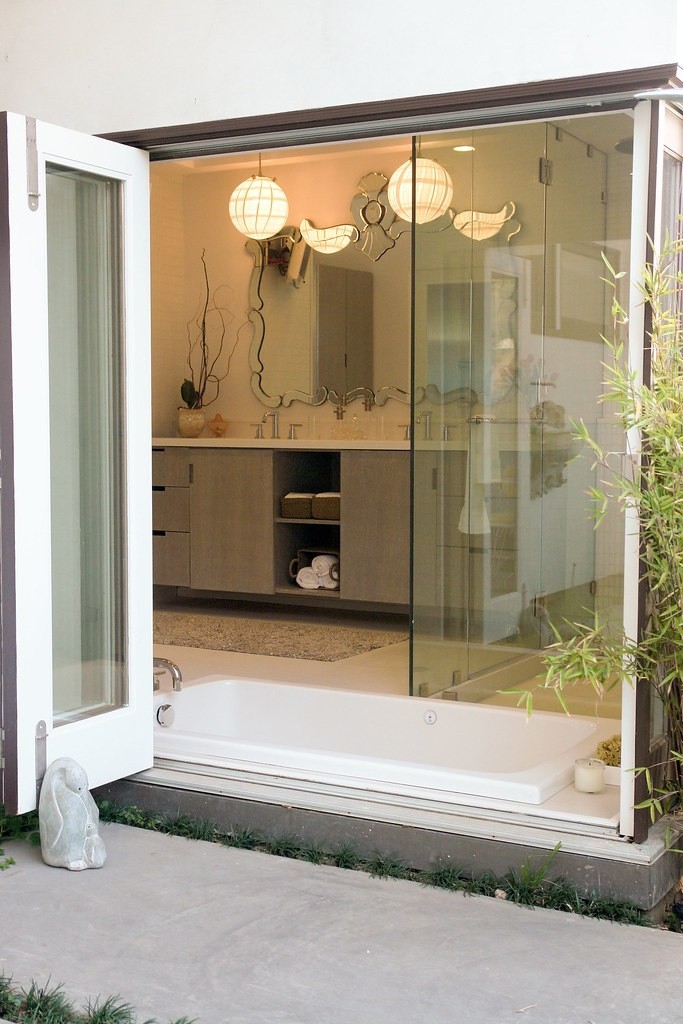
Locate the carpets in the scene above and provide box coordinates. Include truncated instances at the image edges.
[151,601,412,663]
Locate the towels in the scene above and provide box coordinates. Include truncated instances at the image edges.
[457,412,502,534]
[295,554,339,591]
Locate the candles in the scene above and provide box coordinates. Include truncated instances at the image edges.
[573,758,605,793]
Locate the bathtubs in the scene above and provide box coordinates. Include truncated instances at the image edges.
[154,674,622,806]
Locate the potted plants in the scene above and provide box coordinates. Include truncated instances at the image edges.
[176,247,249,437]
[597,731,621,786]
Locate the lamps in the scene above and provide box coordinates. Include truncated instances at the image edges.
[387,135,452,225]
[227,152,289,240]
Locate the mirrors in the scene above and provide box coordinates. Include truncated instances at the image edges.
[245,170,523,420]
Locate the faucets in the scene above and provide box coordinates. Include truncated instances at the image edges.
[261,410,280,439]
[416,411,433,441]
[153,656,183,692]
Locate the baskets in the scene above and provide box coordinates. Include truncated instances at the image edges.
[280,492,311,518]
[312,496,340,520]
[289,546,340,591]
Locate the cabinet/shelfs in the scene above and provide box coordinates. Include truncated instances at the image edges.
[188,447,275,594]
[152,446,190,587]
[435,451,596,618]
[316,263,374,393]
[273,449,339,596]
[338,448,436,607]
